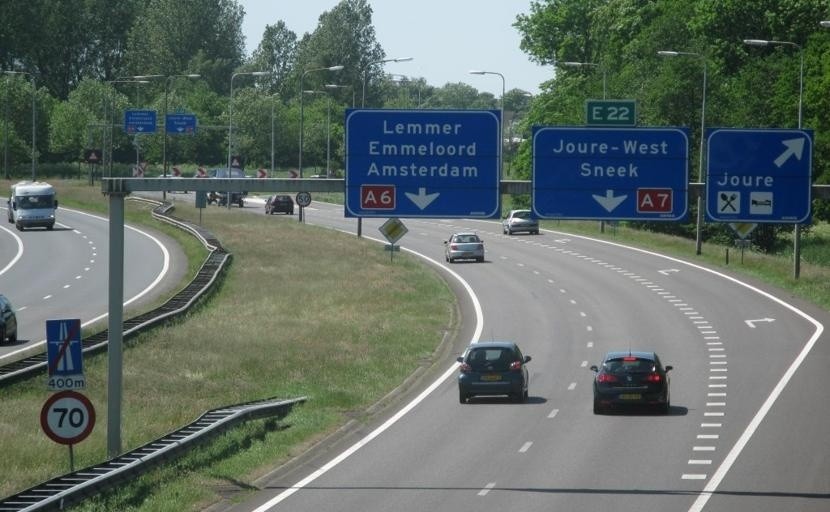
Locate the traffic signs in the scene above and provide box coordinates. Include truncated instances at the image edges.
[296,191,311,207]
[344,107,501,219]
[531,124,689,223]
[40,390,95,444]
[165,113,196,135]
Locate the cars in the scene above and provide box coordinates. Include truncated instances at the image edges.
[444,232,484,262]
[0,294,17,346]
[264,194,294,215]
[456,341,531,402]
[504,209,539,235]
[591,350,673,413]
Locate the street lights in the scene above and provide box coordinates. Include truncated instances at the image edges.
[658,48,708,253]
[163,73,203,201]
[744,38,803,278]
[297,64,355,178]
[227,70,275,177]
[564,60,607,233]
[3,68,36,180]
[357,56,422,238]
[102,74,159,176]
[470,71,505,108]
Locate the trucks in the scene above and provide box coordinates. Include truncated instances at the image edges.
[8,181,58,231]
[206,167,247,205]
